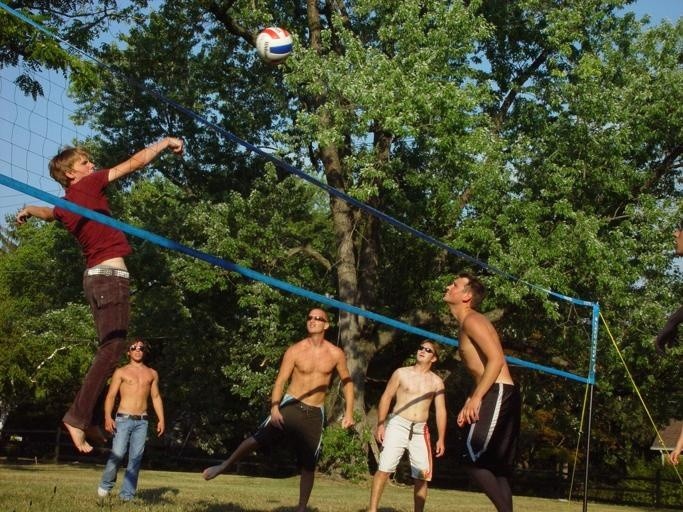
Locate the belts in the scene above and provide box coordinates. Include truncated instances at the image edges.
[116,414,149,420]
[83,269,129,280]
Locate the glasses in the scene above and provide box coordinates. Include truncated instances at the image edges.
[417,346,433,355]
[128,346,144,352]
[306,316,326,322]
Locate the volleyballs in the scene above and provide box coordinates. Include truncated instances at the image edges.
[257,26,292,61]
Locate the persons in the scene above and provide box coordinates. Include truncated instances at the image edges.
[15,137,190,453]
[367,337,448,511]
[667,425,682,467]
[95,341,163,501]
[203,305,356,511]
[442,271,524,511]
